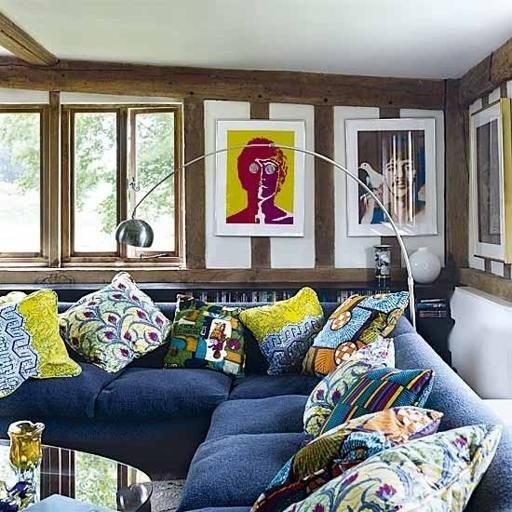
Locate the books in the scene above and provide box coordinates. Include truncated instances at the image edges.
[416,296,450,319]
[188,288,371,303]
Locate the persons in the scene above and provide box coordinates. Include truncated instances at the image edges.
[359,138,427,225]
[226,136,293,224]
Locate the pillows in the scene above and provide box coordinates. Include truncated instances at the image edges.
[239,285,328,378]
[319,367,437,434]
[252,403,445,511]
[284,421,505,512]
[0,289,81,398]
[300,290,412,378]
[59,272,173,373]
[164,293,248,380]
[301,337,397,438]
[0,289,30,305]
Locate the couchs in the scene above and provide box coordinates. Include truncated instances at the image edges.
[0,299,415,477]
[176,332,511,511]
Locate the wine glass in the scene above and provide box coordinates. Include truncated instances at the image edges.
[7,420,45,498]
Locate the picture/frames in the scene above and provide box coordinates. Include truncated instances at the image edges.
[343,117,438,238]
[213,119,306,238]
[468,98,512,276]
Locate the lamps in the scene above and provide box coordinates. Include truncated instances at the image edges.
[114,143,415,327]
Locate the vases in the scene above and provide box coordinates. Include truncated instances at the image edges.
[372,245,392,286]
